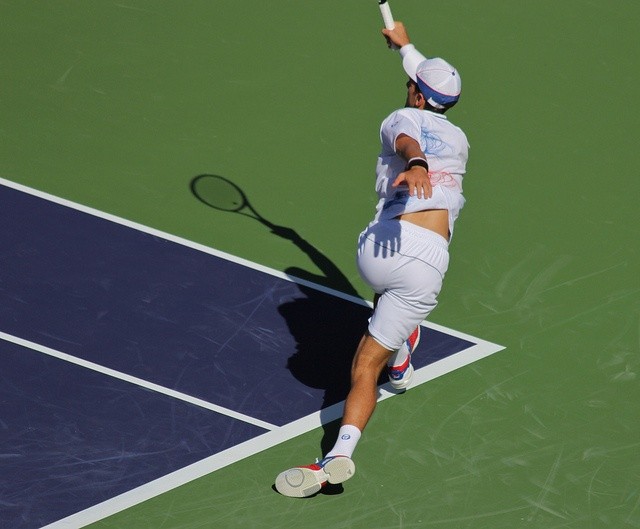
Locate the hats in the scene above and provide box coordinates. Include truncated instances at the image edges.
[403,49,462,108]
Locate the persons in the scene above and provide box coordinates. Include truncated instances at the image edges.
[275,21,470,498]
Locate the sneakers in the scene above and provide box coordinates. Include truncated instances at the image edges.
[388,324,421,388]
[276,456,355,498]
